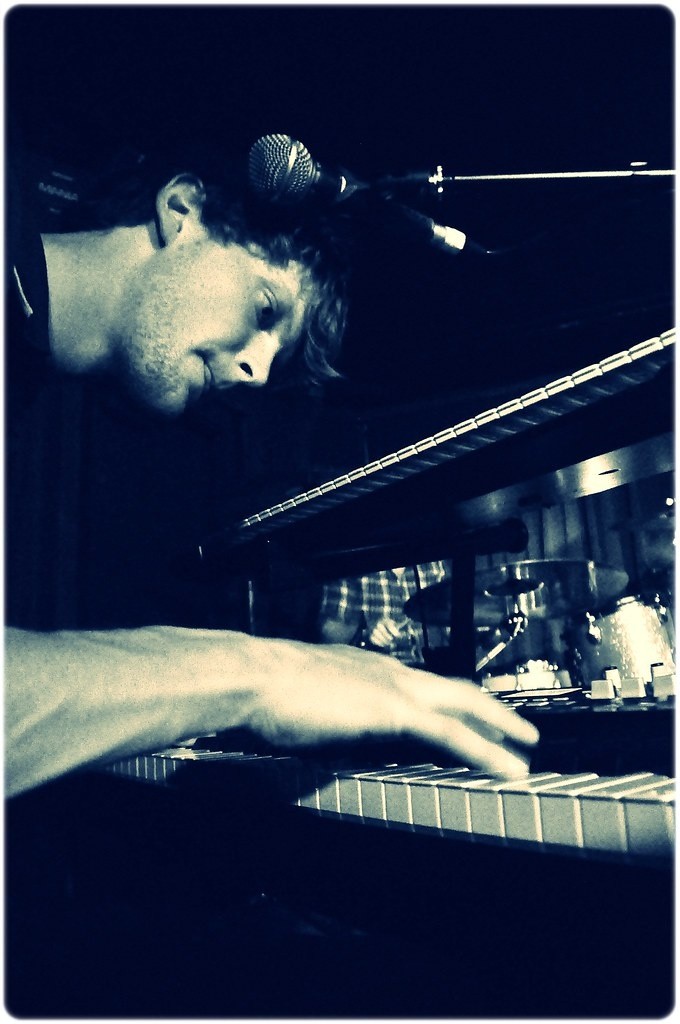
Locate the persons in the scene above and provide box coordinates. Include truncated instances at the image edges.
[319,559,454,667]
[5,172,538,803]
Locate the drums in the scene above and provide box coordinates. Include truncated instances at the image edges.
[560,584,676,688]
[477,656,574,696]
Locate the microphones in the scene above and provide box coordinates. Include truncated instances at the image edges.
[246,134,485,255]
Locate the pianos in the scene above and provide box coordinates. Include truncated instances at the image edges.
[93,663,677,867]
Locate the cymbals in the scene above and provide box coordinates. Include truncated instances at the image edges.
[404,558,628,628]
